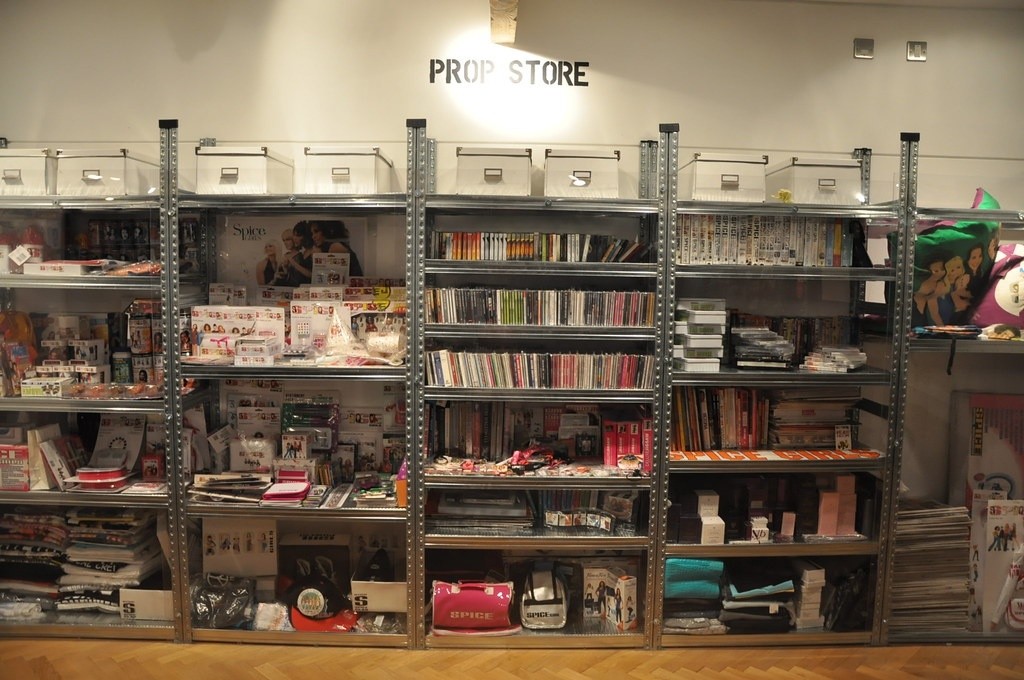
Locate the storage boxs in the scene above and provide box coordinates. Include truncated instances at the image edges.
[119,576,174,622]
[673,151,769,371]
[544,148,621,198]
[456,146,533,197]
[668,482,726,546]
[969,489,1024,632]
[582,562,616,618]
[608,557,641,577]
[765,156,862,205]
[189,251,405,484]
[0,147,58,196]
[278,533,355,575]
[303,144,392,193]
[202,517,278,578]
[55,148,160,196]
[605,566,637,631]
[743,473,858,630]
[194,146,295,195]
[20,298,164,398]
[948,389,1024,511]
[350,545,408,613]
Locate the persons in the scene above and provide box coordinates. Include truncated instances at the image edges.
[228,381,401,486]
[585,581,635,623]
[103,225,142,241]
[206,531,268,556]
[970,521,1021,623]
[180,219,404,354]
[0,327,162,401]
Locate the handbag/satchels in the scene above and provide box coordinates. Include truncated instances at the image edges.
[432,574,515,628]
[519,558,570,628]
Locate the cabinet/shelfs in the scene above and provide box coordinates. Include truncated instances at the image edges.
[669,122,913,650]
[168,119,418,651]
[880,132,1024,648]
[415,118,671,651]
[0,119,184,645]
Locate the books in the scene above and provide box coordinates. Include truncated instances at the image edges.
[424,217,867,478]
[888,497,971,637]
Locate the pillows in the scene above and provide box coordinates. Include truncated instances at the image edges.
[884,187,1001,327]
